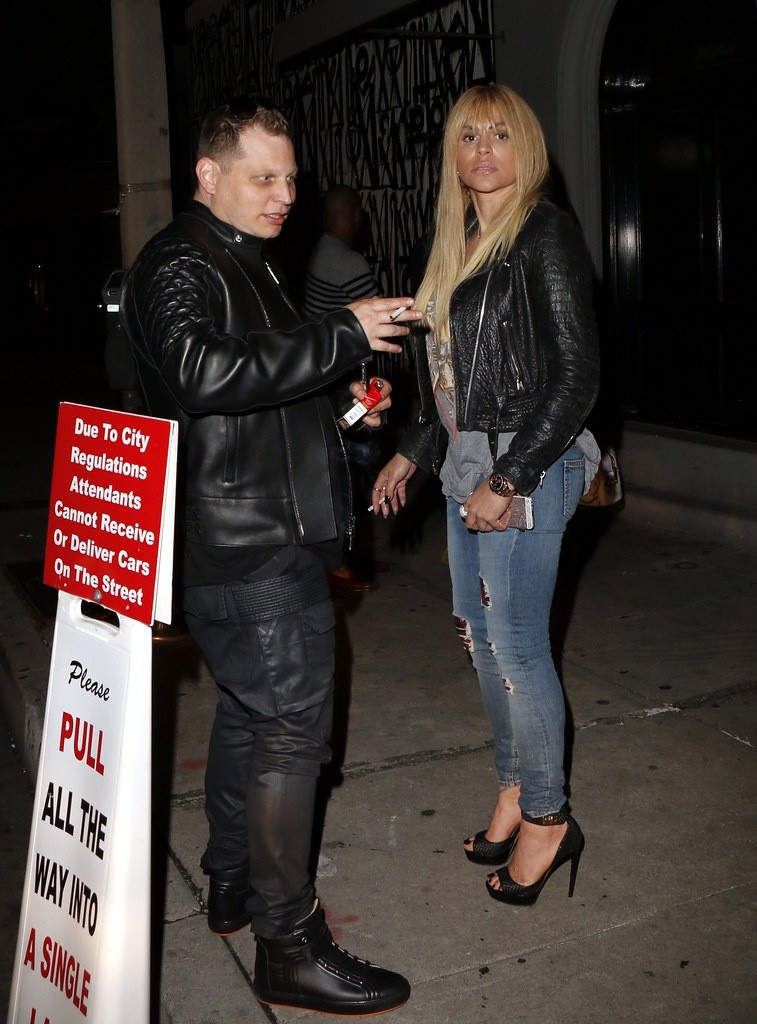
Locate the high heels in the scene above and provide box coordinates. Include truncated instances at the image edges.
[463,815,588,907]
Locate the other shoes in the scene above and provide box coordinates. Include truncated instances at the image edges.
[326,561,387,592]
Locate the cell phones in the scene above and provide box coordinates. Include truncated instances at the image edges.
[499,496,534,529]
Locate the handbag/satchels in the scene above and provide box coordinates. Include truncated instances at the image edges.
[582,445,624,505]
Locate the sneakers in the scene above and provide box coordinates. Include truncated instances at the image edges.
[206,872,254,934]
[254,897,413,1017]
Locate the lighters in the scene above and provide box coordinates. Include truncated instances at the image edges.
[374,379,384,390]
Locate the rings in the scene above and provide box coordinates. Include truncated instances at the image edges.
[372,486,381,492]
[458,505,468,518]
[382,486,385,491]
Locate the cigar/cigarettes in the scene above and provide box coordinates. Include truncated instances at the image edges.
[390,306,407,320]
[368,497,385,511]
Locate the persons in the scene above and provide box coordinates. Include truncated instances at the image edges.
[127,100,422,1017]
[373,81,601,906]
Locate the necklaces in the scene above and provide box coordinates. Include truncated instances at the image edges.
[477,224,481,238]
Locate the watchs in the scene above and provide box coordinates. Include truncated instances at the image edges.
[489,470,516,497]
